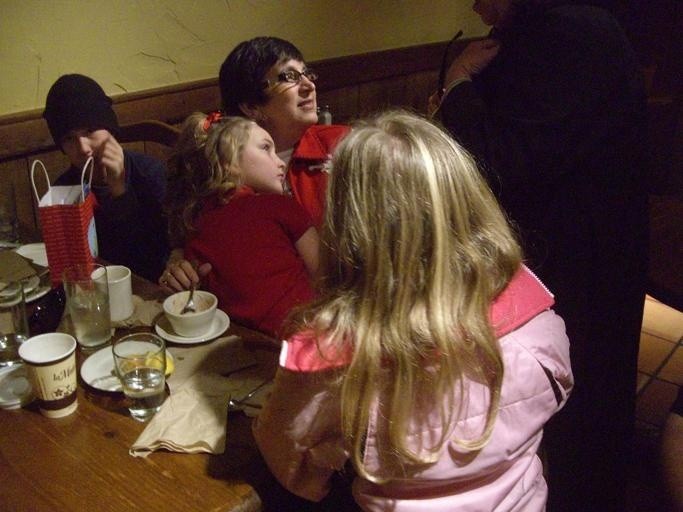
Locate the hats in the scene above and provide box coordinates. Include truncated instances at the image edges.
[42,73,120,155]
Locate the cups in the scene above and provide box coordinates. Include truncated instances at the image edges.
[91,264,135,322]
[0,280,30,367]
[112,331,167,422]
[162,290,217,337]
[18,332,78,420]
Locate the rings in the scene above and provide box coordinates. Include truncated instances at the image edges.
[159,271,171,284]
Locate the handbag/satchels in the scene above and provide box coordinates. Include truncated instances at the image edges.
[29,157,101,291]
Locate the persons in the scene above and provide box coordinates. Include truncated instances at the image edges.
[154,35,358,297]
[39,73,193,290]
[252,107,578,510]
[161,107,329,342]
[420,0,655,512]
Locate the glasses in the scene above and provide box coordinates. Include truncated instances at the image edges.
[259,68,320,89]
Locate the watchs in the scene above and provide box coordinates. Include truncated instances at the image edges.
[432,88,449,108]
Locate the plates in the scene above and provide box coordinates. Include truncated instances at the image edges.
[80,339,173,393]
[156,308,230,345]
[0,264,52,308]
[14,243,49,268]
